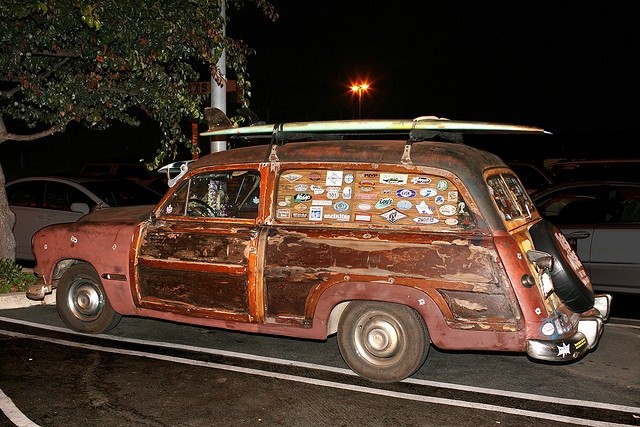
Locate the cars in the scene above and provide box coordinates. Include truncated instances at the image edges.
[528,178,640,294]
[5,171,164,261]
[27,115,611,383]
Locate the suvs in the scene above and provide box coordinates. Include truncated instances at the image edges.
[547,160,640,184]
[504,161,555,195]
